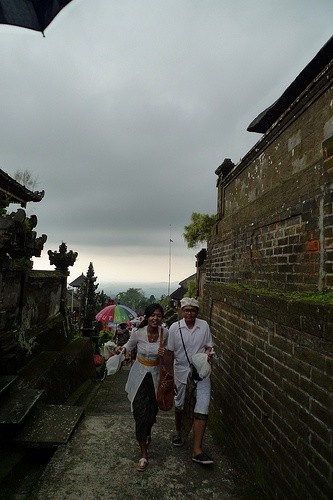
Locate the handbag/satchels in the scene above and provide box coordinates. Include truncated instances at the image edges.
[189,363,203,381]
[157,368,174,411]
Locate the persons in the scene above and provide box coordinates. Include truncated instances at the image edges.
[115,303,169,472]
[114,317,149,371]
[165,297,214,464]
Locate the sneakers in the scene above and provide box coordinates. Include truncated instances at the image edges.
[146,435,151,446]
[136,458,148,471]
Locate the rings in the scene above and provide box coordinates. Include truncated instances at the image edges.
[163,348,165,352]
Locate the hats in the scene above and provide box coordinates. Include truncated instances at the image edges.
[180,297,200,310]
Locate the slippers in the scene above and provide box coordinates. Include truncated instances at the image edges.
[192,452,214,464]
[171,435,183,447]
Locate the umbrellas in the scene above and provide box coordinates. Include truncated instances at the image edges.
[95,302,138,332]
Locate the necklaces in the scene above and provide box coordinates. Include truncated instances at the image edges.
[147,327,158,341]
